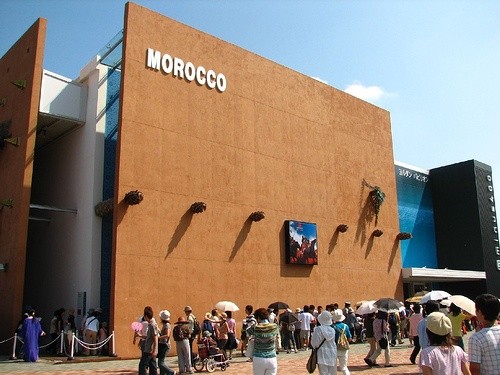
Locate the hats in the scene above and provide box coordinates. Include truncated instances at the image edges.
[204,312,213,320]
[332,309,346,322]
[87,308,94,314]
[218,312,227,320]
[203,331,212,337]
[159,309,170,320]
[317,310,333,326]
[184,306,192,311]
[174,316,189,324]
[97,308,102,313]
[347,307,354,313]
[295,308,301,313]
[425,311,452,335]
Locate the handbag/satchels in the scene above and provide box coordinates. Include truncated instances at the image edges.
[306,349,317,374]
[379,338,388,349]
[158,341,170,352]
[287,324,295,331]
[245,338,255,358]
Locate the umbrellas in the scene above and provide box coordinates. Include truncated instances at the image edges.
[355,300,378,315]
[404,296,425,303]
[215,301,240,312]
[372,297,405,313]
[279,313,301,326]
[414,290,429,296]
[419,290,451,305]
[268,302,290,309]
[448,295,477,316]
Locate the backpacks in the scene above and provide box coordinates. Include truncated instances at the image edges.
[334,324,350,350]
[189,315,201,335]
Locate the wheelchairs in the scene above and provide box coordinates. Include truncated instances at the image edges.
[192,337,230,373]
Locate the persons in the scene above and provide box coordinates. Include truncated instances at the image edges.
[241,301,500,354]
[410,305,424,365]
[172,306,199,375]
[18,309,46,362]
[244,305,258,362]
[311,310,338,375]
[202,305,238,367]
[467,294,500,375]
[132,306,177,375]
[365,311,393,368]
[363,313,379,366]
[15,307,108,359]
[330,309,353,375]
[247,308,278,375]
[418,311,472,375]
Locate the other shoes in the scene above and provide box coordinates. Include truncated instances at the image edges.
[409,357,416,365]
[372,362,379,366]
[385,364,393,367]
[364,358,373,368]
